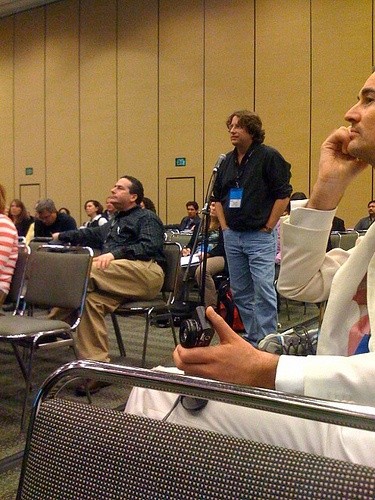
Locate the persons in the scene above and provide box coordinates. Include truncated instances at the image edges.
[0,175,375,394]
[124,68,375,467]
[212,109,294,348]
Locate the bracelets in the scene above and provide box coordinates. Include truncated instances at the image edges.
[264,225,273,232]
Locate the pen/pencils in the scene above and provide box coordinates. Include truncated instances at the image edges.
[50,232,53,236]
[184,246,190,256]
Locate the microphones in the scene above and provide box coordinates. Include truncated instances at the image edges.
[212,154,226,174]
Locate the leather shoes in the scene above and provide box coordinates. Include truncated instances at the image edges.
[78,376,106,392]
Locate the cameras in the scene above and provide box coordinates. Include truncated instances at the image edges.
[179,306,214,349]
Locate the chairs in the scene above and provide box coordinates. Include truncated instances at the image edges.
[0,225,375,500]
